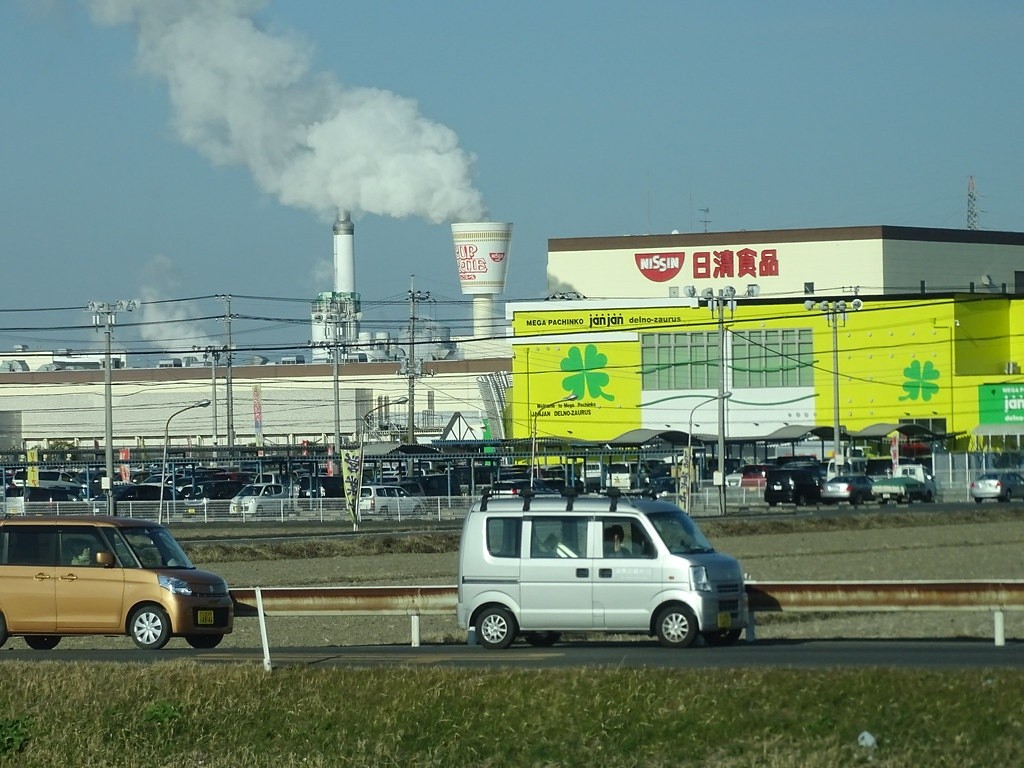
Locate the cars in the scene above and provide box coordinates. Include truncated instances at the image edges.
[0,455,932,524]
[971,472,1024,504]
[818,474,880,505]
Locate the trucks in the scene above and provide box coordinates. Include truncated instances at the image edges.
[869,461,935,505]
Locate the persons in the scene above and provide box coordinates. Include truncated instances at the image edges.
[72,539,98,567]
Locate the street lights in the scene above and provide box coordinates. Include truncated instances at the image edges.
[529,394,580,489]
[352,396,411,529]
[685,390,733,517]
[153,399,215,527]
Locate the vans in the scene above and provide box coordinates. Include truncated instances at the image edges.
[358,485,426,518]
[762,469,818,508]
[227,481,290,518]
[0,513,239,649]
[451,483,751,650]
